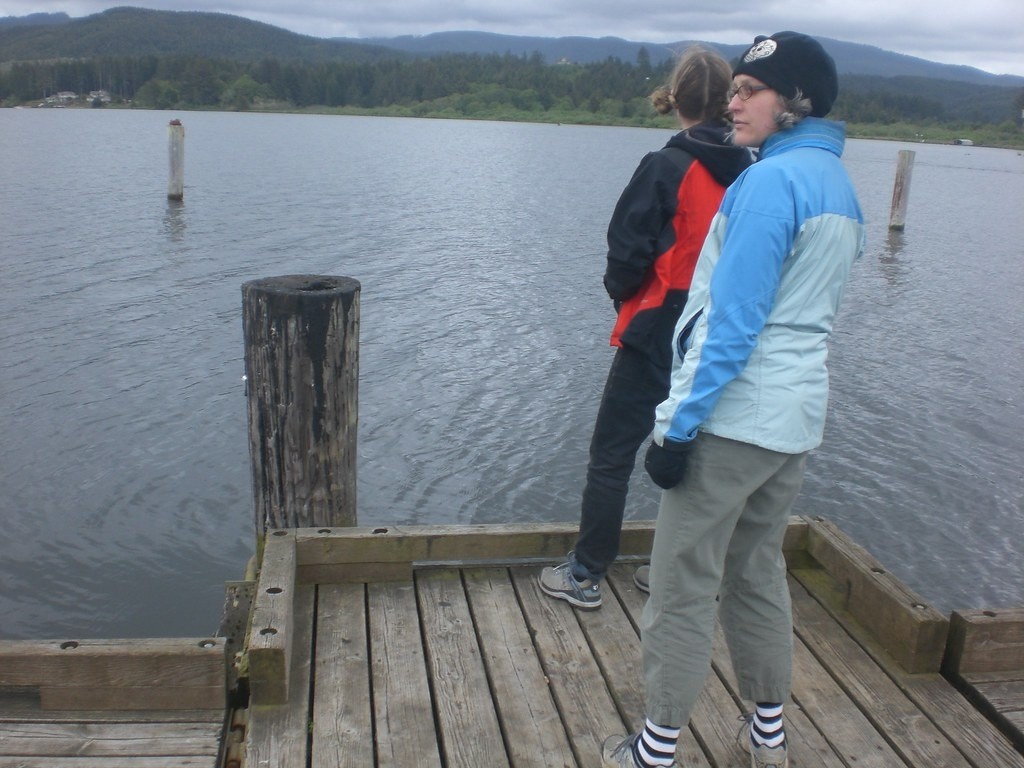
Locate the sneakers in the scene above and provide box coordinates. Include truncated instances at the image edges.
[633,565,650,593]
[538,564,603,612]
[600,735,677,767]
[740,722,791,767]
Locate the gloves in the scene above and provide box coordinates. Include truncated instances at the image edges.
[644,440,685,490]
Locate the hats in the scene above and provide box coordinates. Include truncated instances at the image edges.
[732,30,838,116]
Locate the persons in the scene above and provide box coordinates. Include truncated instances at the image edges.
[600,30,868,768]
[537,49,765,607]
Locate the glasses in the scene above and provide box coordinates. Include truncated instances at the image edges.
[726,86,771,105]
